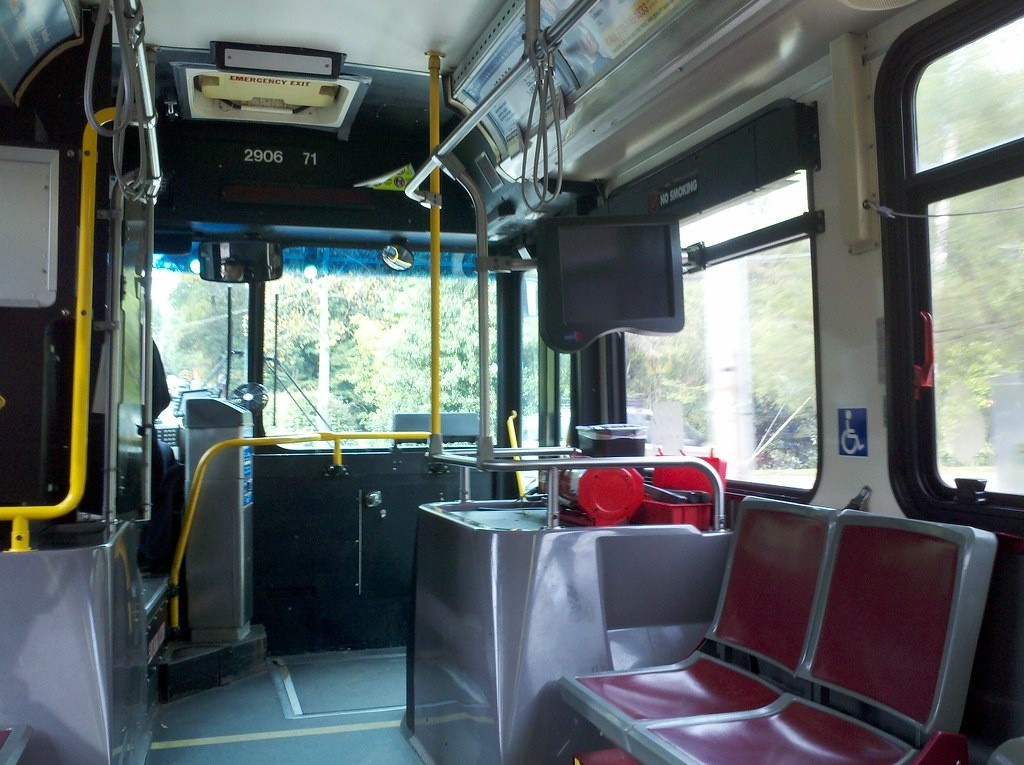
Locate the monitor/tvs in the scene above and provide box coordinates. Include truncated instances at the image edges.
[536,213,685,353]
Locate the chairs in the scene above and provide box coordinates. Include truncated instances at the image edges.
[552,496,842,751]
[628,509,999,765]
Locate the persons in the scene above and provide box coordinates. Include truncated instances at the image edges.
[221,259,254,282]
[136,323,183,574]
